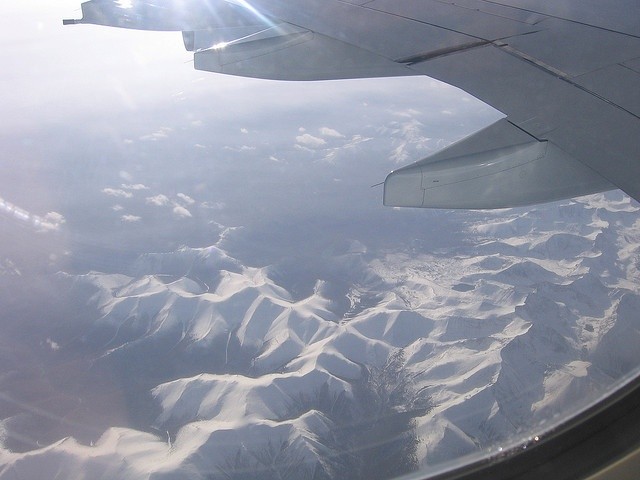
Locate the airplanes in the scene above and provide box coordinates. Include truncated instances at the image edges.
[51,0,640,480]
